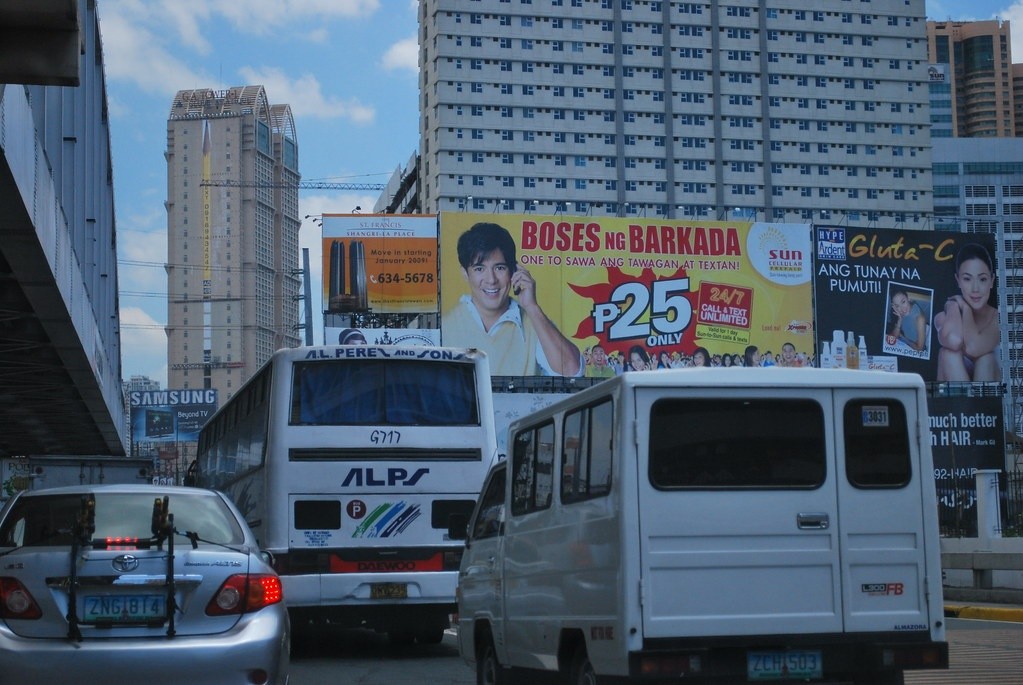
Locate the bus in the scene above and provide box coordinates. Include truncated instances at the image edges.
[181,344,507,651]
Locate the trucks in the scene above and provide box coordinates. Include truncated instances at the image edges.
[0,454,26,511]
[22,454,155,492]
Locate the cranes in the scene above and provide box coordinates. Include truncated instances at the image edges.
[199,170,393,191]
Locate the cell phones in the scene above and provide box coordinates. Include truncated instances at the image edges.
[511,260,522,296]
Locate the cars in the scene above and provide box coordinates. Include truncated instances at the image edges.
[0,480,291,685]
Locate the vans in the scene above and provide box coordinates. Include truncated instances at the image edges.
[453,366,950,685]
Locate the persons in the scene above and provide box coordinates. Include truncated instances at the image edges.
[583,342,814,377]
[934,243,1001,382]
[444,222,584,378]
[338,329,367,346]
[890,292,930,350]
